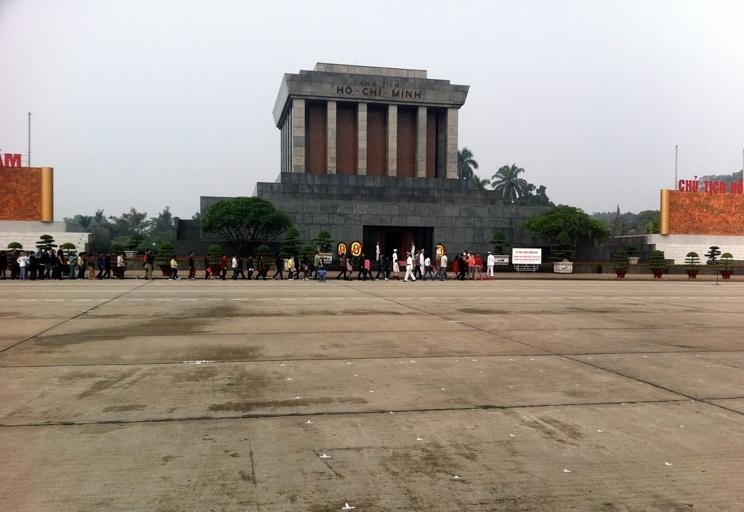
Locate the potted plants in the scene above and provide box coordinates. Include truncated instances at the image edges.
[612,245,736,279]
[58,235,317,276]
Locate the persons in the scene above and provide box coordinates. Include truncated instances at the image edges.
[187,251,195,280]
[142,249,153,280]
[170,255,181,280]
[204,250,321,281]
[0,250,126,280]
[335,249,494,282]
